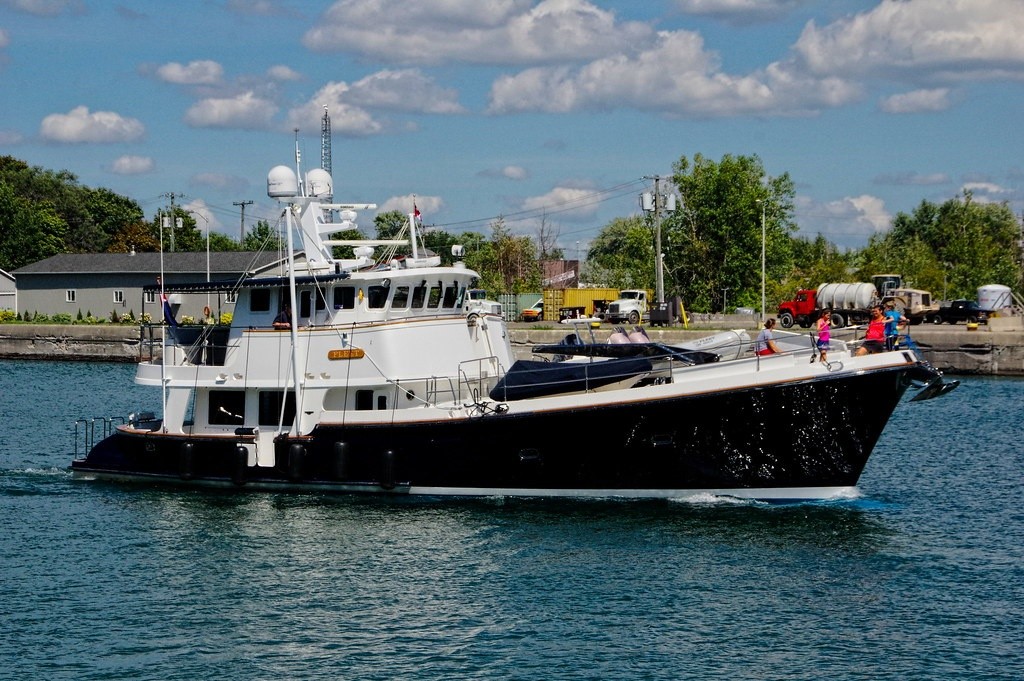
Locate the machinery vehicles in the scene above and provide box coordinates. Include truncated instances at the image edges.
[777,281,879,329]
[872,274,940,326]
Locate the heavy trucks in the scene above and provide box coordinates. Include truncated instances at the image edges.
[606,290,692,324]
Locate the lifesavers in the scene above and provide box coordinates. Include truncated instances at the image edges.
[203,306,211,315]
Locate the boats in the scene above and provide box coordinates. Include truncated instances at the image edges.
[67,131,961,510]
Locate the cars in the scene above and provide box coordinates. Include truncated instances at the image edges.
[523,298,544,321]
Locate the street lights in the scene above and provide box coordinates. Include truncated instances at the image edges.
[185,209,210,319]
[754,199,765,323]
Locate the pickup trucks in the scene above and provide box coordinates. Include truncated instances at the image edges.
[925,300,997,325]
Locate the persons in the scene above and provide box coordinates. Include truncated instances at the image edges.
[856,305,894,355]
[755,318,783,356]
[272,304,291,329]
[882,301,907,351]
[817,309,831,362]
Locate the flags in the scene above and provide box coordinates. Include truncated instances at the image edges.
[157,279,178,327]
[414,204,422,222]
